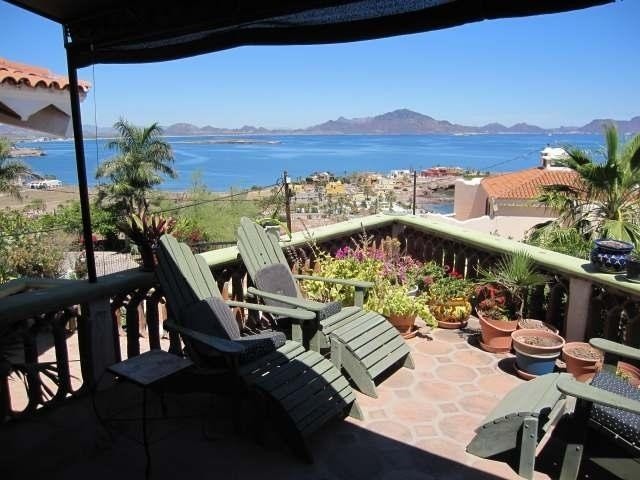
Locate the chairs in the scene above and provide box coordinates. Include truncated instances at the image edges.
[236,216,416,400]
[154,233,364,465]
[464,336,639,479]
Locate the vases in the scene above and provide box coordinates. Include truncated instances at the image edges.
[137,247,158,272]
[384,238,640,392]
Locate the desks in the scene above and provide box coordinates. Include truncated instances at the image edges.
[91,349,226,480]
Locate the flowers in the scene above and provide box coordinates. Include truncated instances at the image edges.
[115,212,177,250]
